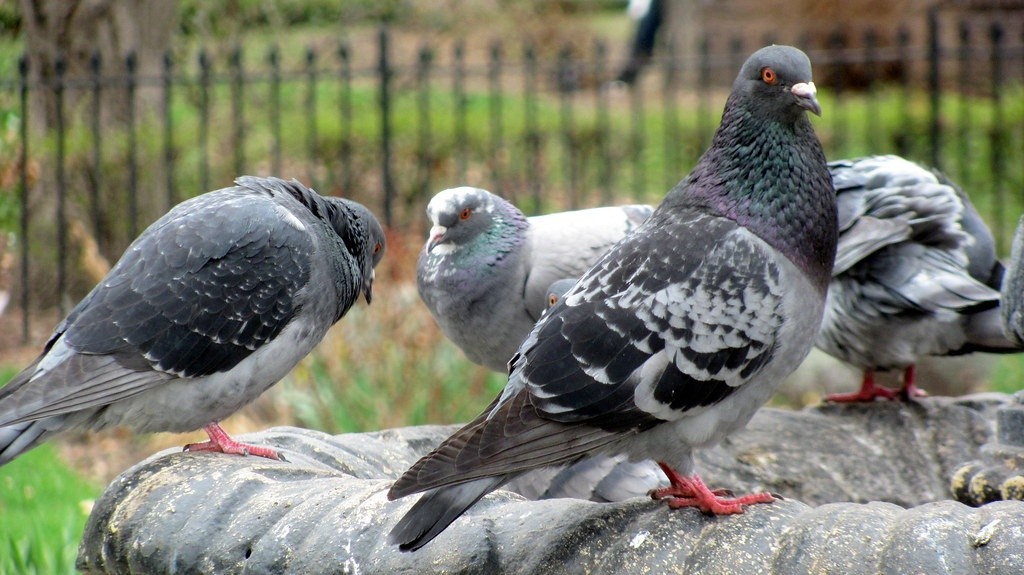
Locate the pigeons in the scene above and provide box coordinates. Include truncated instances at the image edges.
[380,44,1008,555]
[0,175,383,466]
